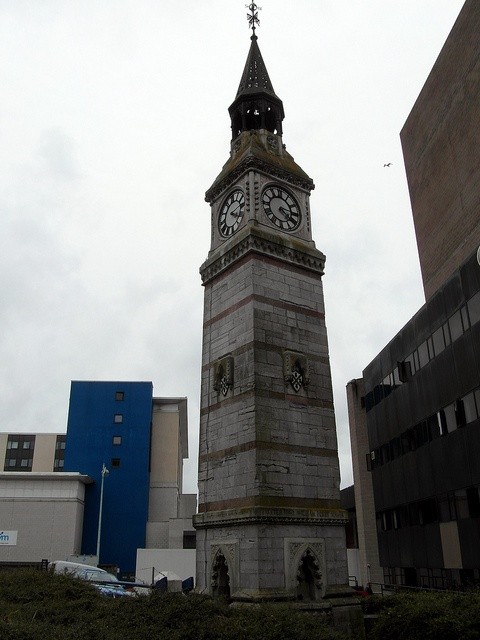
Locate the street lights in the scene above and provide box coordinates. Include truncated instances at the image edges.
[97,463,109,567]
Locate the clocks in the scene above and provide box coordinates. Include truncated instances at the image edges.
[261,186,301,231]
[217,190,245,237]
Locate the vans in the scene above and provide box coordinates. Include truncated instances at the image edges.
[48,560,134,598]
